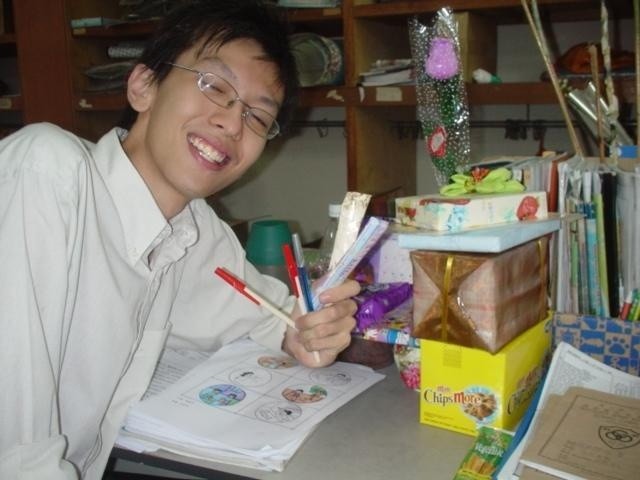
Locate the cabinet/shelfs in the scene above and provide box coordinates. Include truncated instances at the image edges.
[1,0,166,143]
[286,1,640,229]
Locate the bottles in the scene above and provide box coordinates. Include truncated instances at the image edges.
[242,216,296,296]
[319,200,342,271]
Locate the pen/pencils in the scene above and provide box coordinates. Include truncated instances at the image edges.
[618,277,640,321]
[213,233,322,365]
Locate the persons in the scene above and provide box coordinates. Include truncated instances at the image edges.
[0,0,362,480]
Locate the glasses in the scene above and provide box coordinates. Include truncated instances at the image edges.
[157,60,283,139]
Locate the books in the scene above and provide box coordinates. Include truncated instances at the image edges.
[397,218,562,254]
[70,16,126,28]
[362,68,415,88]
[456,151,640,321]
